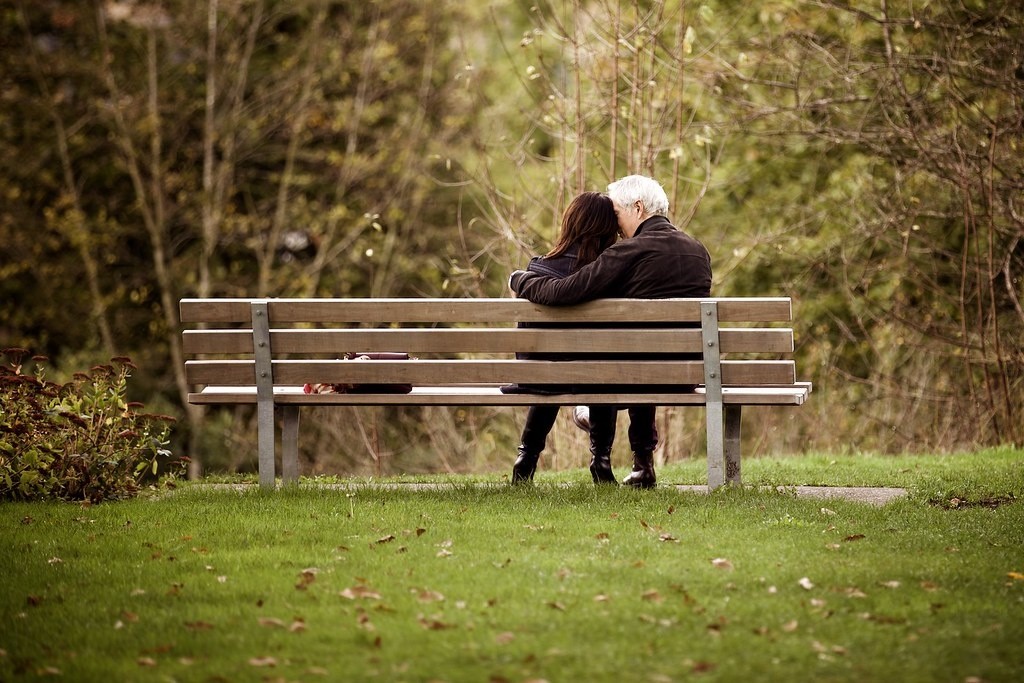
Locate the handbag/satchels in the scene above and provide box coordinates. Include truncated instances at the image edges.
[333,353,412,394]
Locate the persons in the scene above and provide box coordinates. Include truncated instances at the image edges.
[509,175,712,491]
[501,192,621,484]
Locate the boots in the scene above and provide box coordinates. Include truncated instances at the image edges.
[573,405,590,438]
[622,447,657,490]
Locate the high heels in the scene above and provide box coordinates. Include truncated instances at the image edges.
[512,446,541,486]
[590,448,619,486]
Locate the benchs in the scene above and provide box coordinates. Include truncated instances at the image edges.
[179,297,814,493]
